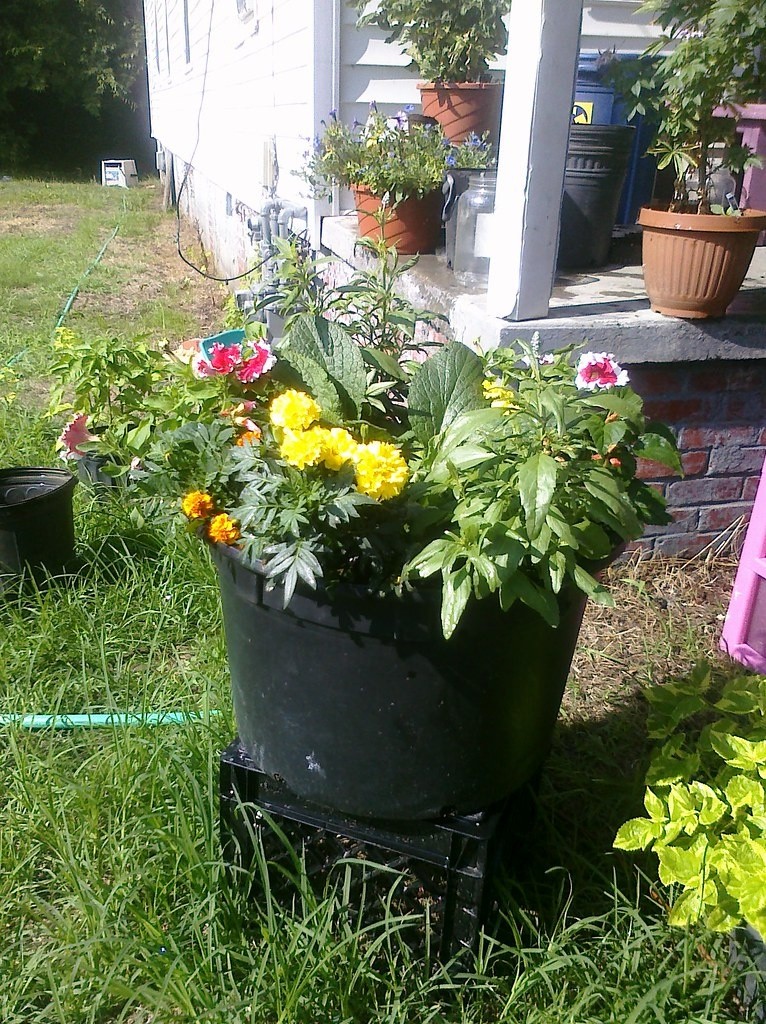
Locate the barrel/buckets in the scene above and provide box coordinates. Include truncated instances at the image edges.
[0,466,79,586]
[555,52,668,270]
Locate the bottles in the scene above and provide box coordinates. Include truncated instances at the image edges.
[452,177,496,290]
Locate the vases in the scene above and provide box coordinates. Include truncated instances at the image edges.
[205,538,600,819]
[351,184,444,254]
[0,467,80,611]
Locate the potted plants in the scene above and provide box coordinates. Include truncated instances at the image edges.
[596,0,766,319]
[344,0,513,147]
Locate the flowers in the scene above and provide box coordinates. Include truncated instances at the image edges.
[291,101,497,217]
[45,189,687,642]
